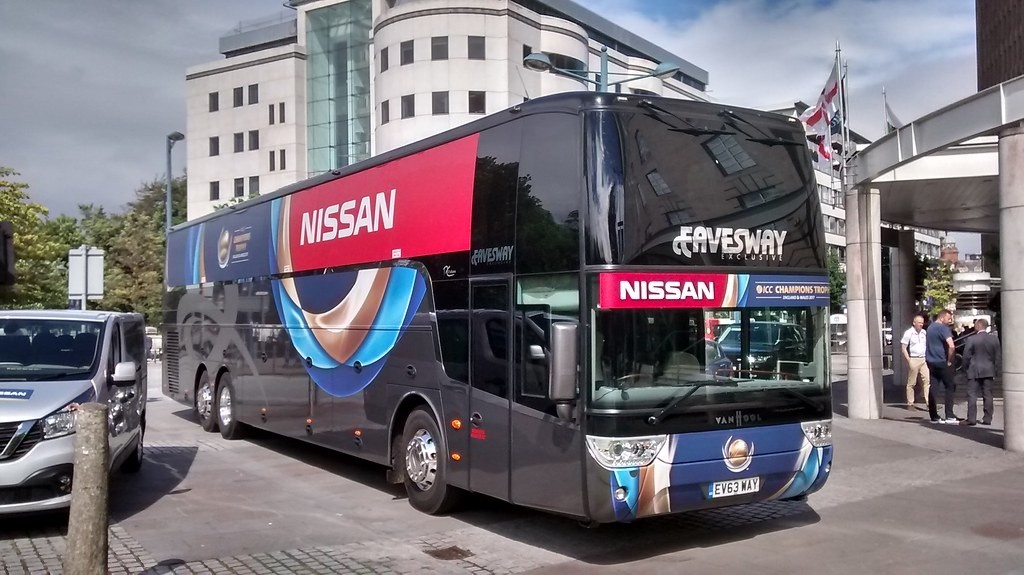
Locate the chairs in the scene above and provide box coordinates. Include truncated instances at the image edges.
[69,333,98,367]
[664,352,700,381]
[31,333,61,355]
[58,334,75,354]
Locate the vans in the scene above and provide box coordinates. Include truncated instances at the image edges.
[0,309,146,514]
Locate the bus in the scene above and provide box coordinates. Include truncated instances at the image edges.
[162,89,834,529]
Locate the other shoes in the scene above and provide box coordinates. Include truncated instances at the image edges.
[930,416,946,424]
[945,418,965,423]
[907,405,916,411]
[977,419,991,425]
[927,406,942,412]
[959,420,976,426]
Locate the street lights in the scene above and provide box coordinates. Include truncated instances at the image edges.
[521,44,683,94]
[165,131,186,227]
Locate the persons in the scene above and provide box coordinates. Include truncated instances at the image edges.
[925,309,964,424]
[959,319,1000,426]
[953,319,977,355]
[901,315,943,411]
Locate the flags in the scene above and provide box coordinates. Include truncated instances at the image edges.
[800,54,849,172]
[884,102,903,134]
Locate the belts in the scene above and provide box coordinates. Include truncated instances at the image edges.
[910,356,920,358]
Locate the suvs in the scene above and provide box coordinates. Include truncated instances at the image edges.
[715,322,810,379]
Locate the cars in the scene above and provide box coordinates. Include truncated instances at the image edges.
[706,339,735,378]
